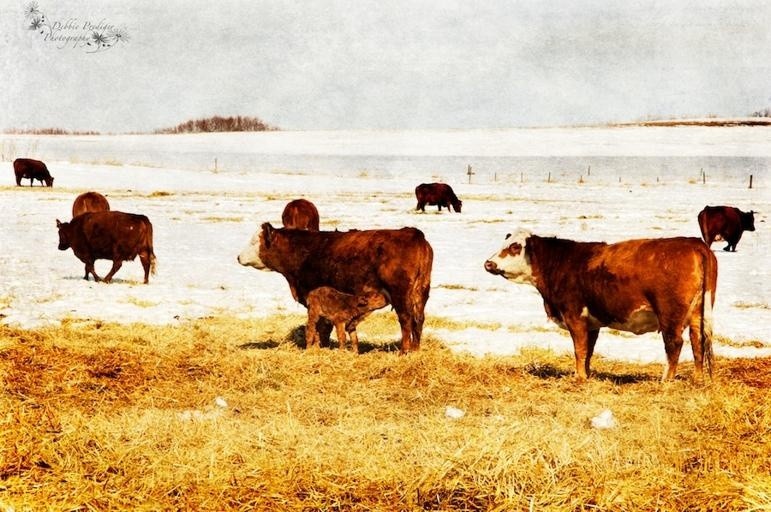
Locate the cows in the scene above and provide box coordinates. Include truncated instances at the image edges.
[415,183,462,213]
[282,199,319,230]
[72,192,110,218]
[56,211,157,284]
[237,221,433,356]
[305,286,390,353]
[698,205,754,252]
[484,228,718,384]
[14,158,54,188]
